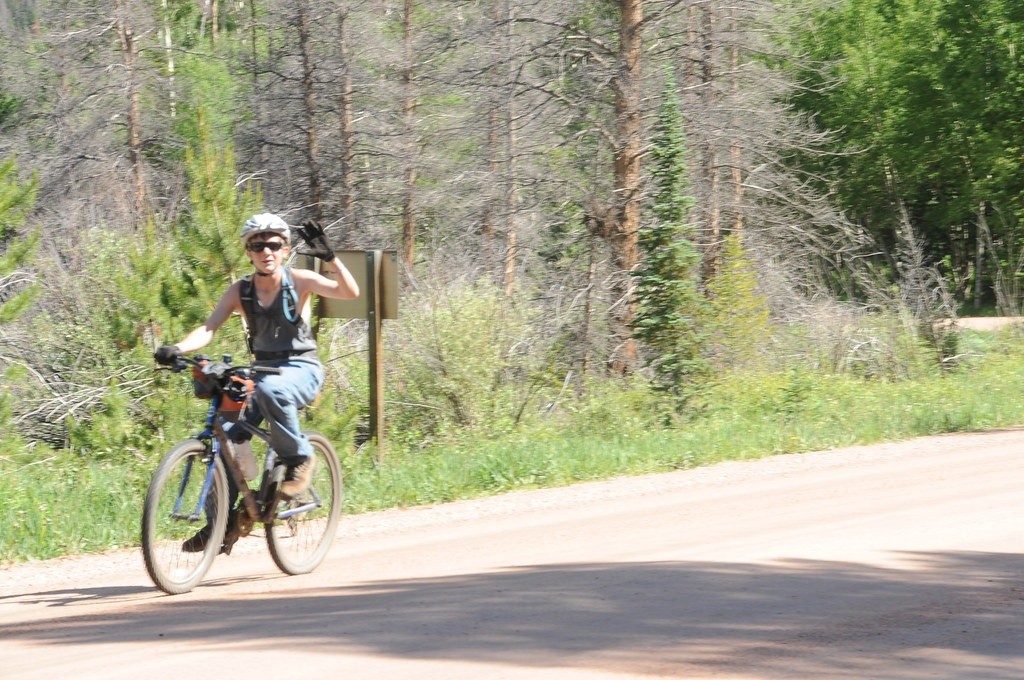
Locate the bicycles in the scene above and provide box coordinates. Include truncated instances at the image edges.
[141,353,344,595]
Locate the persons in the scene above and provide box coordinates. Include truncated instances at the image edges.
[152,211,363,555]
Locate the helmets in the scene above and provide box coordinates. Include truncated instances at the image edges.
[240,210,293,246]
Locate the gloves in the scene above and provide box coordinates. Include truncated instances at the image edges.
[152,345,182,365]
[296,221,336,263]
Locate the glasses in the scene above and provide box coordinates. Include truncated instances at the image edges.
[246,240,287,254]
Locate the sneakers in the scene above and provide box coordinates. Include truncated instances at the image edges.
[279,453,322,501]
[182,521,240,552]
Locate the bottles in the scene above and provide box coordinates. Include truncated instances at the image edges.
[234,434,259,481]
